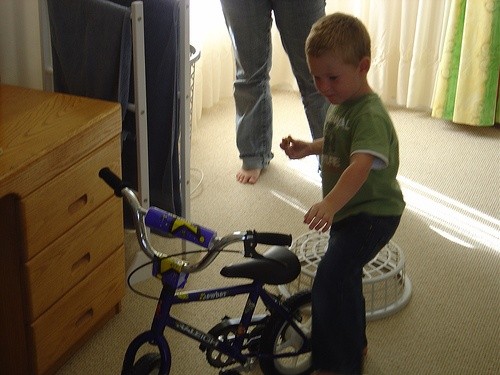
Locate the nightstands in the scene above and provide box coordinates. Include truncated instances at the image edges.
[0,84,126,375]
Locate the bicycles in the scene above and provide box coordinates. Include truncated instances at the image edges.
[96,168,320,375]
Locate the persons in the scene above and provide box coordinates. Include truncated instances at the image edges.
[221,0,331,184]
[279,12,406,375]
